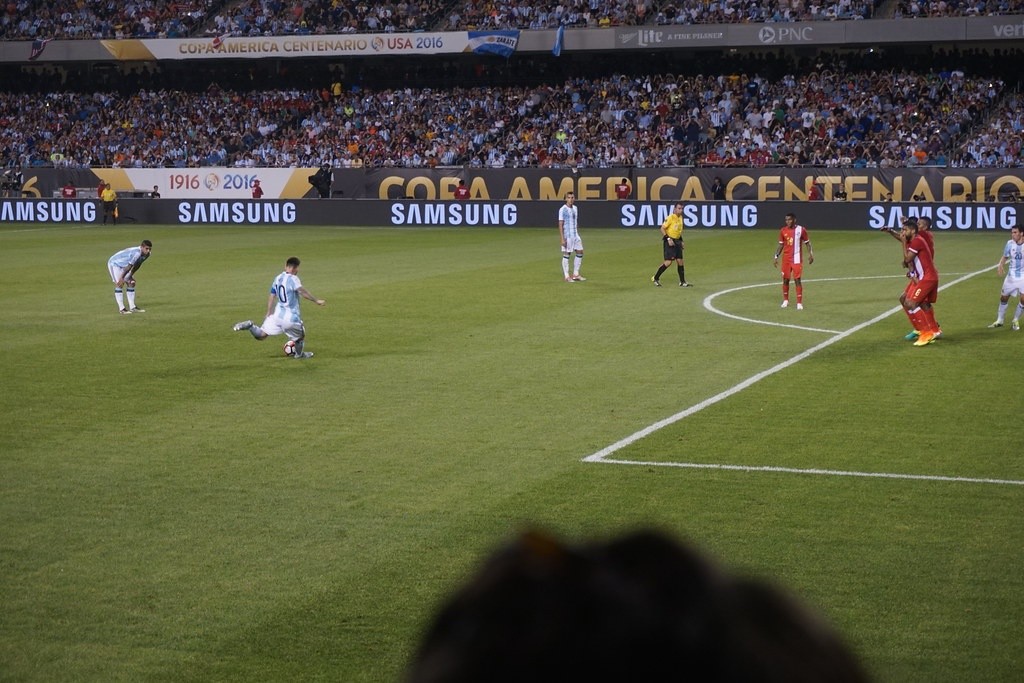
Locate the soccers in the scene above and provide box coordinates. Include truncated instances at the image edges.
[283,341,296,357]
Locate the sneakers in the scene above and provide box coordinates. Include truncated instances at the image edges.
[988,321,1004,329]
[566,277,574,283]
[680,282,692,287]
[120,308,131,314]
[131,306,144,312]
[234,320,253,331]
[651,275,662,286]
[573,275,585,281]
[294,352,313,358]
[905,327,942,346]
[781,300,788,308]
[1012,320,1020,330]
[797,303,803,310]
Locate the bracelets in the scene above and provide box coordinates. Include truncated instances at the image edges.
[909,272,915,278]
[116,202,118,203]
[773,254,778,259]
[667,238,670,240]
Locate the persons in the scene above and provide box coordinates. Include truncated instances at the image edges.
[773,213,814,310]
[879,215,942,346]
[987,222,1024,330]
[407,519,866,683]
[559,192,587,283]
[0,0,1024,227]
[233,257,327,360]
[108,240,154,315]
[650,200,690,287]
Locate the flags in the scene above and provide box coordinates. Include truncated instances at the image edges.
[468,30,520,58]
[552,26,563,58]
[212,33,231,48]
[27,37,53,59]
